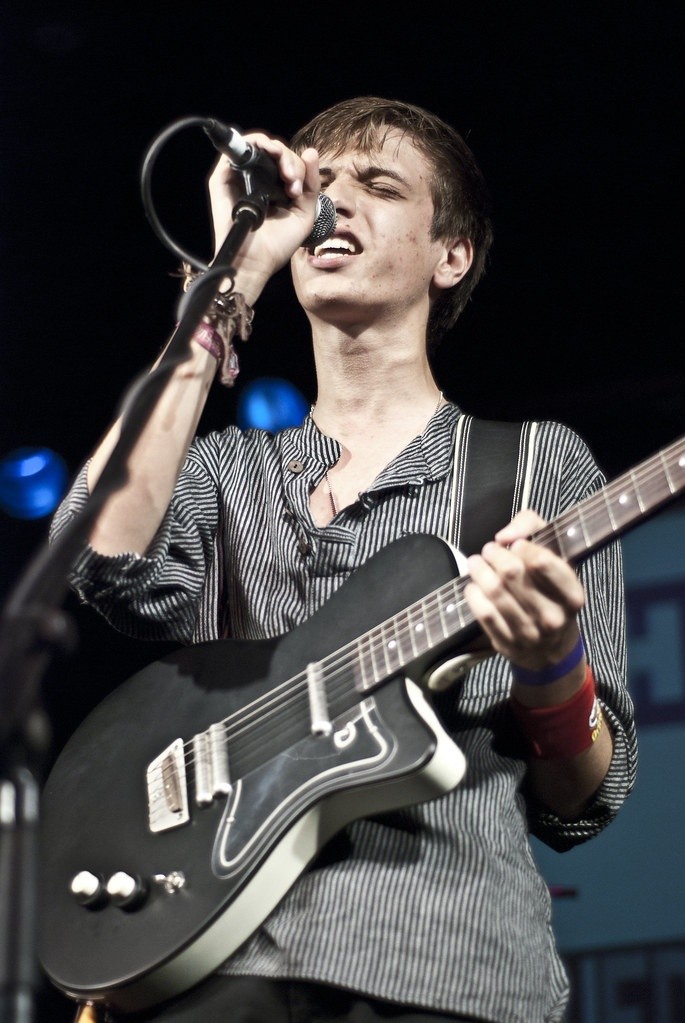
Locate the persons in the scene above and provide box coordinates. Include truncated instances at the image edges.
[52,98,638,1023]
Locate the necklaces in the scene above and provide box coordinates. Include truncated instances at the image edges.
[323,470,338,517]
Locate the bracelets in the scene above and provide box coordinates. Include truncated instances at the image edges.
[512,666,604,761]
[194,322,239,385]
[183,268,255,333]
[510,635,584,687]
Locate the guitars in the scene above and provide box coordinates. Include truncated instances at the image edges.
[31,434,685,1012]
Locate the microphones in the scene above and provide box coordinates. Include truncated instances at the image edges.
[204,119,338,249]
